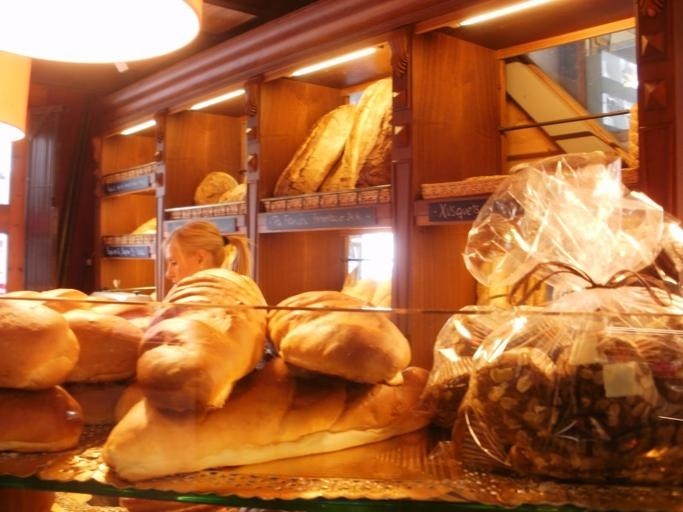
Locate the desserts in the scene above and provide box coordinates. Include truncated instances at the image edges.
[430,308,682,472]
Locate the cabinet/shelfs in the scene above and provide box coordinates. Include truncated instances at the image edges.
[51,1,682,367]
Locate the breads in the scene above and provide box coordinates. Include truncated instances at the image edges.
[218,184,247,202]
[2,289,89,300]
[320,76,392,192]
[63,309,144,383]
[274,104,356,196]
[266,291,412,384]
[136,269,269,413]
[103,355,432,480]
[1,299,80,390]
[1,385,83,451]
[194,171,239,204]
[60,383,128,424]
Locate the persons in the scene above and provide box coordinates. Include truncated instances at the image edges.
[162,218,254,284]
